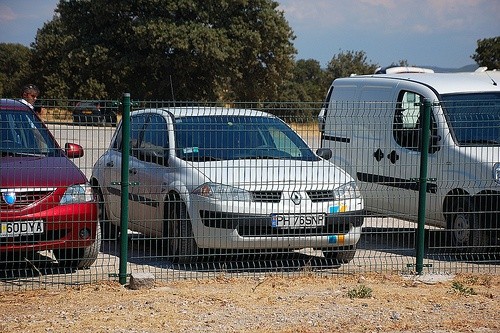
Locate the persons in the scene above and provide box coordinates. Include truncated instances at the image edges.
[20,84,40,111]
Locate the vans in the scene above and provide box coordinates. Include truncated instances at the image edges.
[318,71,500,258]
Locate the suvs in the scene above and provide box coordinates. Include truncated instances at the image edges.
[71,98,117,128]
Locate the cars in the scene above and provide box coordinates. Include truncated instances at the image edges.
[90,107,365,264]
[0,97,102,271]
